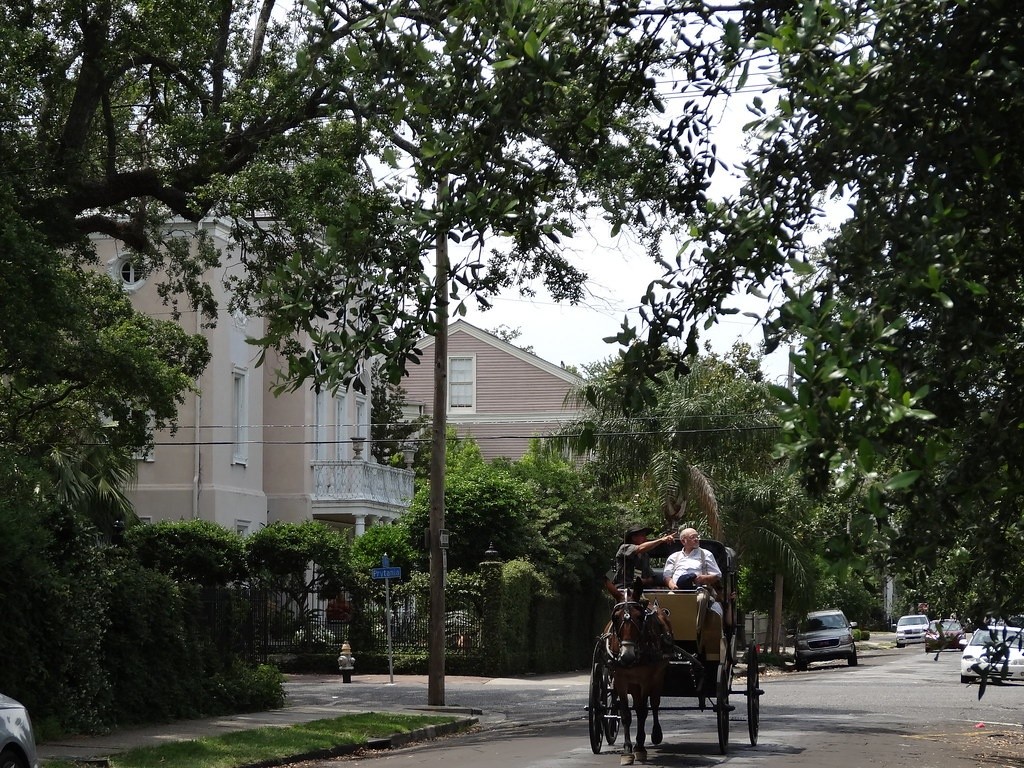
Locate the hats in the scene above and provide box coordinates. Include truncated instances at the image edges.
[626,523,655,537]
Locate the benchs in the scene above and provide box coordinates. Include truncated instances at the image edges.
[640,539,737,638]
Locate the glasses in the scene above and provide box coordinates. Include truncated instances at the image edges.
[683,535,700,539]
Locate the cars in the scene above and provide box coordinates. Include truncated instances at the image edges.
[892,614,929,648]
[923,619,967,652]
[0,693,40,768]
[959,626,1024,684]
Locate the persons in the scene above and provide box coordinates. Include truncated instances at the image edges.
[606,527,677,588]
[664,528,722,642]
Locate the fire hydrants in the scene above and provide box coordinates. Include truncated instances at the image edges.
[338,638,356,684]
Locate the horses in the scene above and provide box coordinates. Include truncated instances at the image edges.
[602,576,673,765]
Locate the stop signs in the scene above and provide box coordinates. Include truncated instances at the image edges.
[918,602,928,613]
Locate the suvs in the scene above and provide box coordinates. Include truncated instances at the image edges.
[787,608,858,671]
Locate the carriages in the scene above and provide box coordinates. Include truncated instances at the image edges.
[583,536,765,754]
